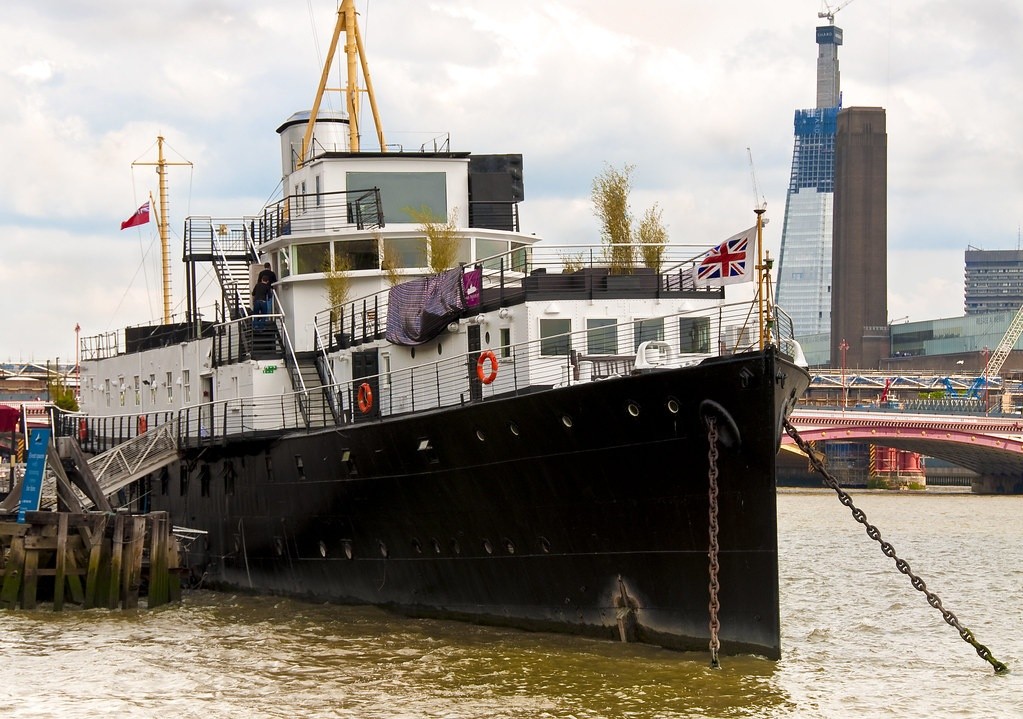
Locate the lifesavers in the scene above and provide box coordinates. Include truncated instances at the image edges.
[79,420,87,438]
[477,350,498,384]
[357,383,373,414]
[138,416,146,434]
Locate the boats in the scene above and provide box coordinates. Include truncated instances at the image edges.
[44,0,829,665]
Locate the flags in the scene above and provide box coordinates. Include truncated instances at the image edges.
[692,224,761,286]
[120,202,150,229]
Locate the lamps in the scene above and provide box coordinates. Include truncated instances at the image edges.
[143,380,150,385]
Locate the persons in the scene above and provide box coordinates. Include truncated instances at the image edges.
[280,263,289,276]
[253,263,276,331]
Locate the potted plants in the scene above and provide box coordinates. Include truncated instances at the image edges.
[318,247,351,349]
[632,201,669,292]
[591,160,642,291]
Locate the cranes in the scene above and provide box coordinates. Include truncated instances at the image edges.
[964,303,1023,411]
